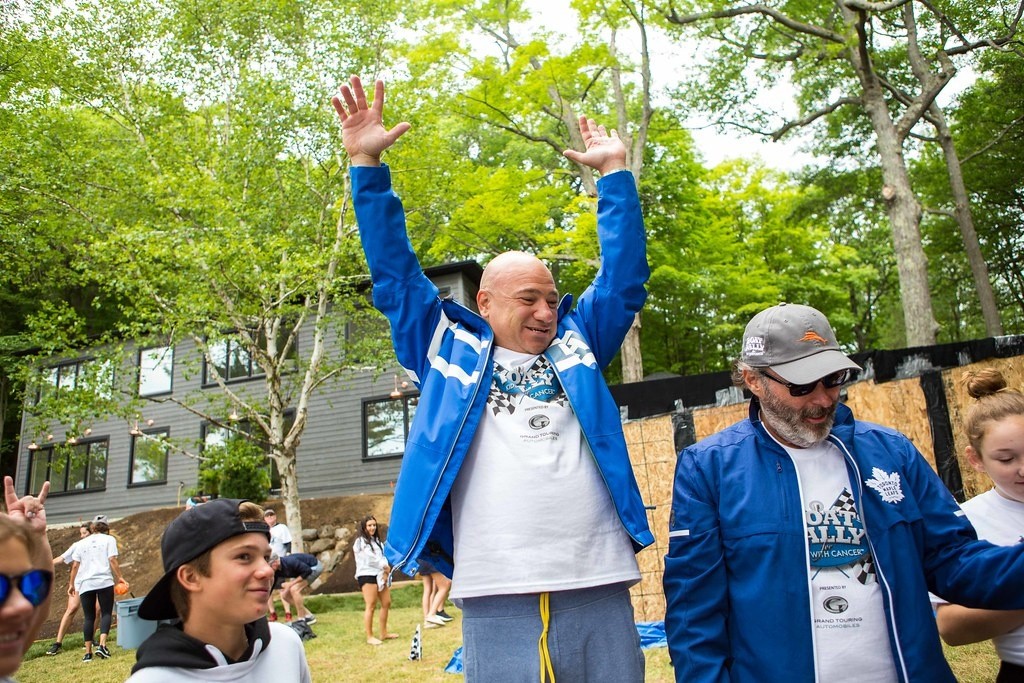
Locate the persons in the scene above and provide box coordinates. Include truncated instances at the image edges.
[123,499,311,683]
[416,559,454,628]
[46,515,130,662]
[0,476,54,683]
[661,303,1024,683]
[264,509,323,625]
[331,76,654,683]
[353,515,399,645]
[186,497,204,509]
[929,371,1023,682]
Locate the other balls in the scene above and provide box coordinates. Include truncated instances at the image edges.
[115,582,129,596]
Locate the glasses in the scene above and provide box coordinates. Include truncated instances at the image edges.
[266,513,274,516]
[0,569,56,611]
[754,368,848,397]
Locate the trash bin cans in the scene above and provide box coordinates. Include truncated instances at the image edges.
[115,597,158,648]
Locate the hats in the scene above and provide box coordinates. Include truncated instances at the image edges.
[742,302,864,385]
[263,508,275,515]
[136,498,271,622]
[92,514,110,526]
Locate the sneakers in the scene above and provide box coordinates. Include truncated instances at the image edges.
[90,641,100,646]
[83,642,94,649]
[285,612,292,622]
[270,612,278,622]
[81,652,93,662]
[304,613,317,625]
[299,618,307,625]
[46,643,62,655]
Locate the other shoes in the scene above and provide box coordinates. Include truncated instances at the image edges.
[423,622,440,629]
[95,645,111,659]
[426,613,446,626]
[436,609,453,622]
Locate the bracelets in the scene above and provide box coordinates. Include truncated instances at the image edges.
[602,168,622,176]
